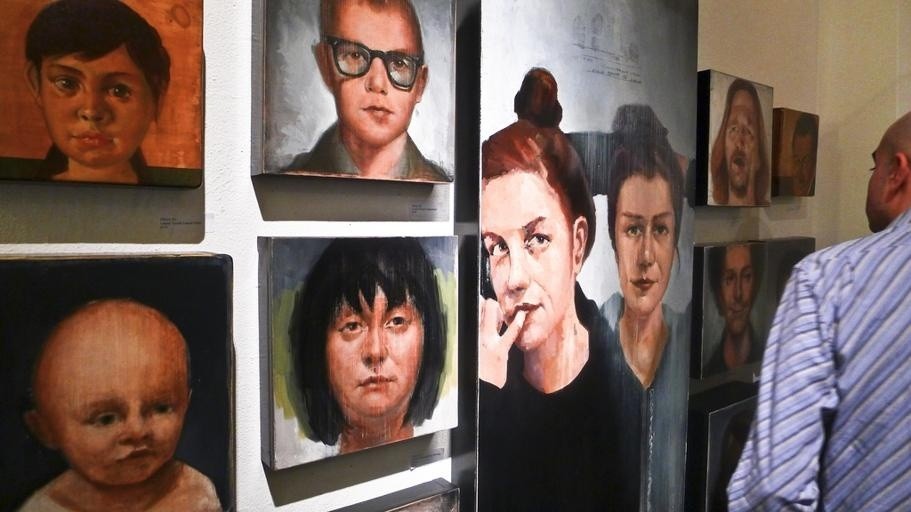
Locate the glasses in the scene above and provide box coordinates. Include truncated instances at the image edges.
[322,33,424,89]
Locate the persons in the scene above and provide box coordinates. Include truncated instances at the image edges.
[478,68,645,510]
[703,240,765,378]
[286,238,448,460]
[710,78,769,206]
[725,108,910,512]
[283,0,450,185]
[790,112,817,196]
[776,246,804,309]
[22,0,170,185]
[598,105,685,511]
[19,297,224,511]
